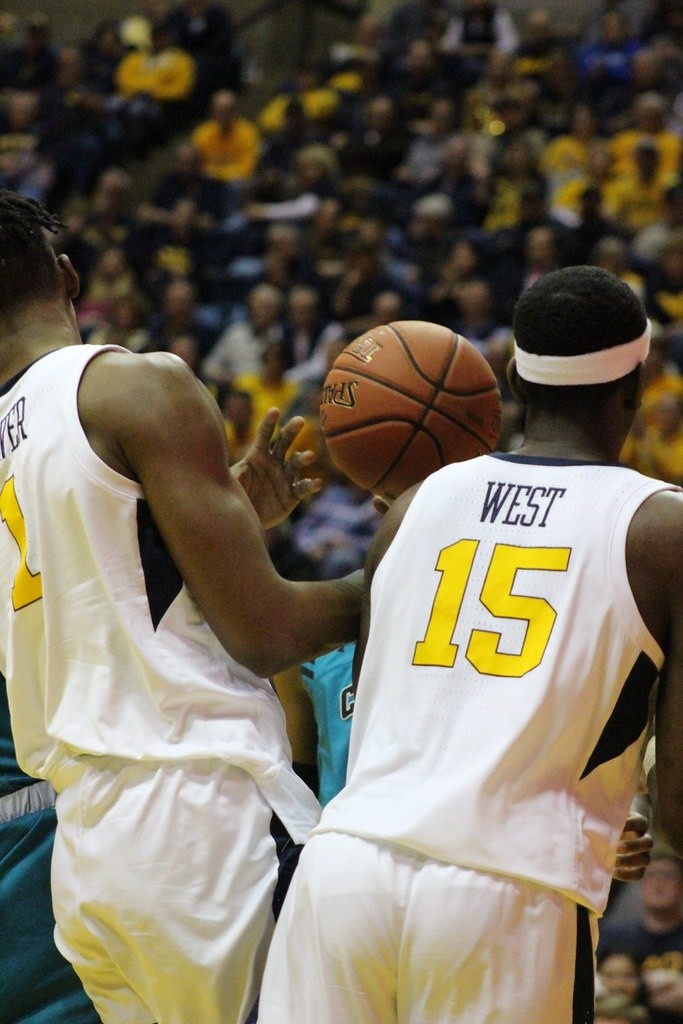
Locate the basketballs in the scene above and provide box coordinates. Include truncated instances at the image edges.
[316,318,507,500]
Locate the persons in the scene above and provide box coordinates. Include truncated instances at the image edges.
[0,0,683,1024]
